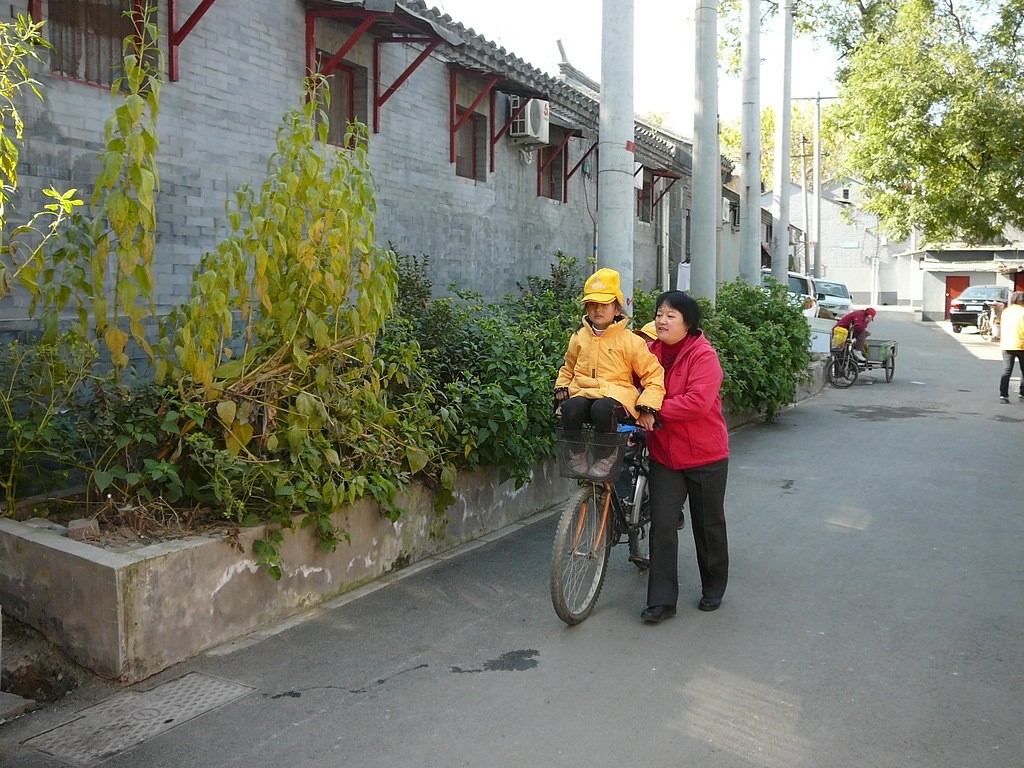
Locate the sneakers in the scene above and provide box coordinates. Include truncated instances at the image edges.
[587,445,619,477]
[569,451,589,476]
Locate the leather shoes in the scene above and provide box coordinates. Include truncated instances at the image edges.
[640,604,676,622]
[698,593,721,611]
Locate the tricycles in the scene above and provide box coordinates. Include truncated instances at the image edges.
[826,320,898,389]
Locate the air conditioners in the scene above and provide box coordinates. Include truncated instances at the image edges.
[509,94,551,145]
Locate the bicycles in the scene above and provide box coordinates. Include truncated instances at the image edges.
[976,310,992,340]
[551,399,661,625]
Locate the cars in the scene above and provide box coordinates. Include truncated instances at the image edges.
[950,286,1014,333]
[760,265,854,322]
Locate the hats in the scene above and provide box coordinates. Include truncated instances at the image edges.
[580,268,623,307]
[631,320,658,340]
[866,307,876,321]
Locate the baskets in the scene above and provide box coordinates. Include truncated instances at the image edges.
[554,424,632,483]
[829,333,846,352]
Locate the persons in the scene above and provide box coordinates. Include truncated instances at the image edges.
[831,308,875,384]
[633,291,729,622]
[554,267,666,476]
[998,291,1024,403]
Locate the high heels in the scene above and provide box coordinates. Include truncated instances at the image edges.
[1019,394,1024,402]
[1000,396,1010,404]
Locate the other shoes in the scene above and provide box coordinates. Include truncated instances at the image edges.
[852,348,866,361]
